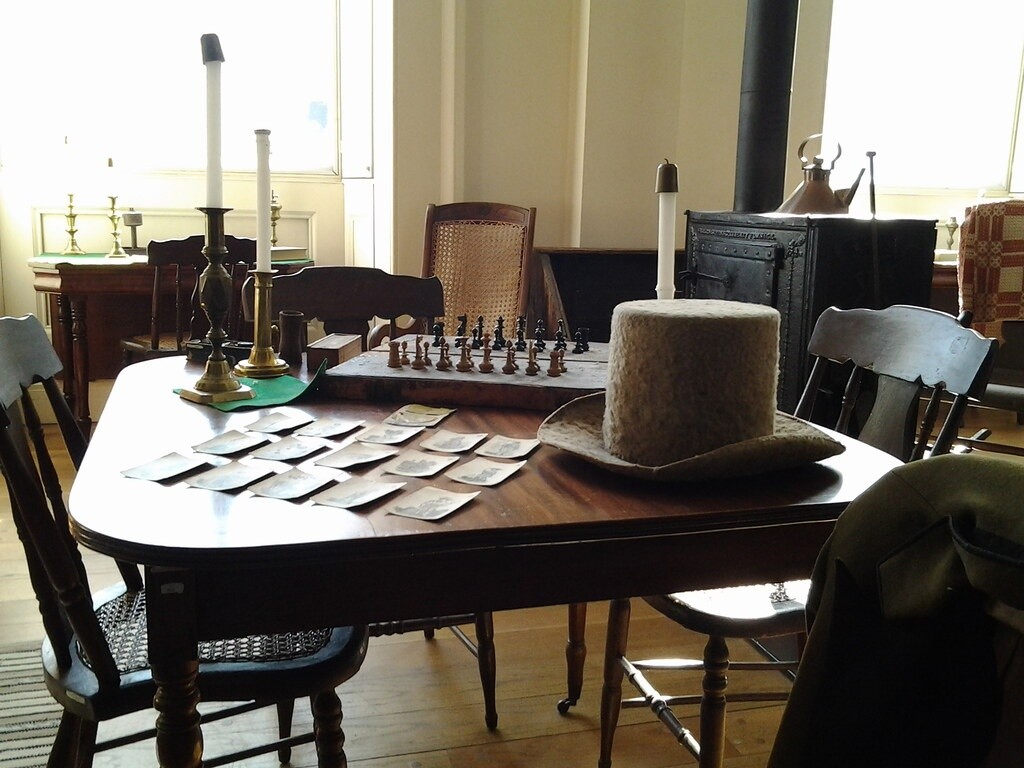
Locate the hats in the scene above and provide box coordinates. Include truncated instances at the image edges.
[537,299,846,483]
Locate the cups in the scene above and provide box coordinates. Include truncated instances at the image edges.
[279,310,304,368]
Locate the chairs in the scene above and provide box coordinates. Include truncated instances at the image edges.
[751,452,1024,768]
[0,312,369,768]
[121,234,256,370]
[955,196,1024,347]
[421,200,538,337]
[239,266,503,734]
[594,305,1001,768]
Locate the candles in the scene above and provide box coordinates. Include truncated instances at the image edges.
[654,161,678,299]
[255,128,272,270]
[64,137,74,192]
[200,32,224,209]
[108,158,116,196]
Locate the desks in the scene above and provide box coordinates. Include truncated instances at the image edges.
[49,352,908,768]
[31,252,315,432]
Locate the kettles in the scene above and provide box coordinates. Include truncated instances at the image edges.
[773,134,867,215]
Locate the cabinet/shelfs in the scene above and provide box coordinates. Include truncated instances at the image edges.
[683,210,939,411]
